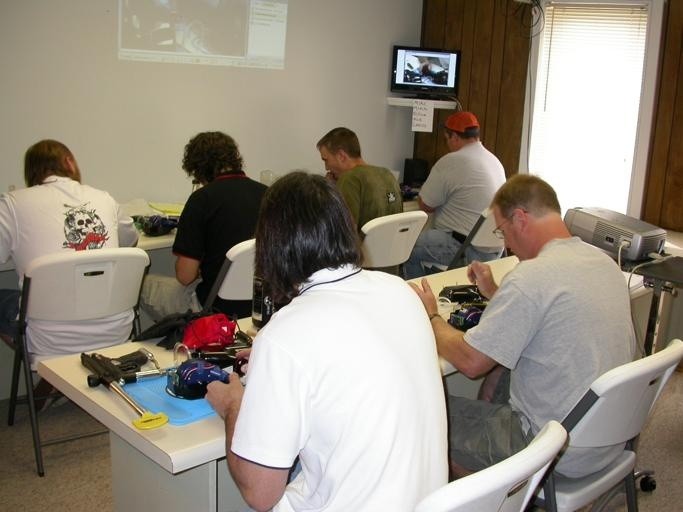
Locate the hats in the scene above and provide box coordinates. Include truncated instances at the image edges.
[445,111,480,133]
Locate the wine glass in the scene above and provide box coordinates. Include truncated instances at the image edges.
[252,269,276,327]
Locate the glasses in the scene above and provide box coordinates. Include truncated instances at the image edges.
[492,210,529,237]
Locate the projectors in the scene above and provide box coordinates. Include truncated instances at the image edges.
[564,206,668,262]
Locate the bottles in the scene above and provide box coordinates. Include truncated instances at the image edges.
[191,179,202,193]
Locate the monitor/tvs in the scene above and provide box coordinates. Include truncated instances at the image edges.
[390,46,462,101]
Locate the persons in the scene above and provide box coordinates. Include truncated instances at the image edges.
[206,167,450,512]
[0,138,138,413]
[138,131,271,335]
[315,127,404,277]
[402,111,507,281]
[408,174,639,481]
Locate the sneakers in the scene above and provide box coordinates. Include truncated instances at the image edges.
[31,377,69,413]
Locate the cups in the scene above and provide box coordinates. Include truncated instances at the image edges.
[260,169,273,186]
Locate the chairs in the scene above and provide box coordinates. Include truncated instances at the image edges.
[361,210,428,275]
[528,339,683,512]
[422,207,505,275]
[412,420,567,511]
[0,248,150,476]
[202,239,256,312]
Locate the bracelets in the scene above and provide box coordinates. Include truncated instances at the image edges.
[429,313,444,320]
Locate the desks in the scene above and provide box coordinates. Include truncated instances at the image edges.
[38,230,683,511]
[0,186,422,272]
